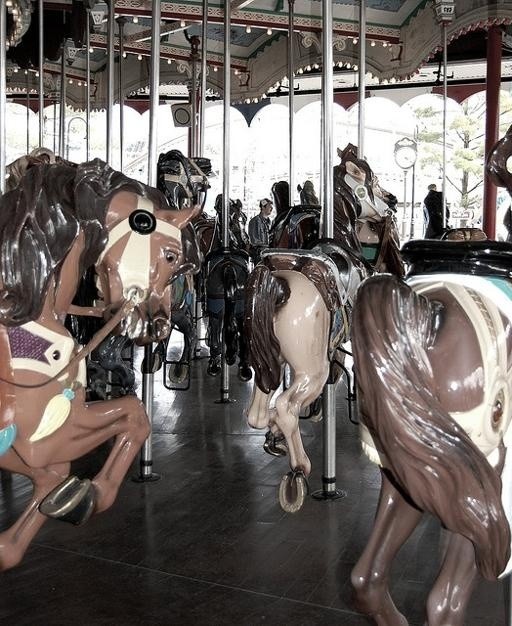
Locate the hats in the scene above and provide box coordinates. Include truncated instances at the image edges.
[259,198,274,207]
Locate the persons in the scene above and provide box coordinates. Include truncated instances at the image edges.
[300,181,319,205]
[424,184,450,240]
[249,198,273,264]
